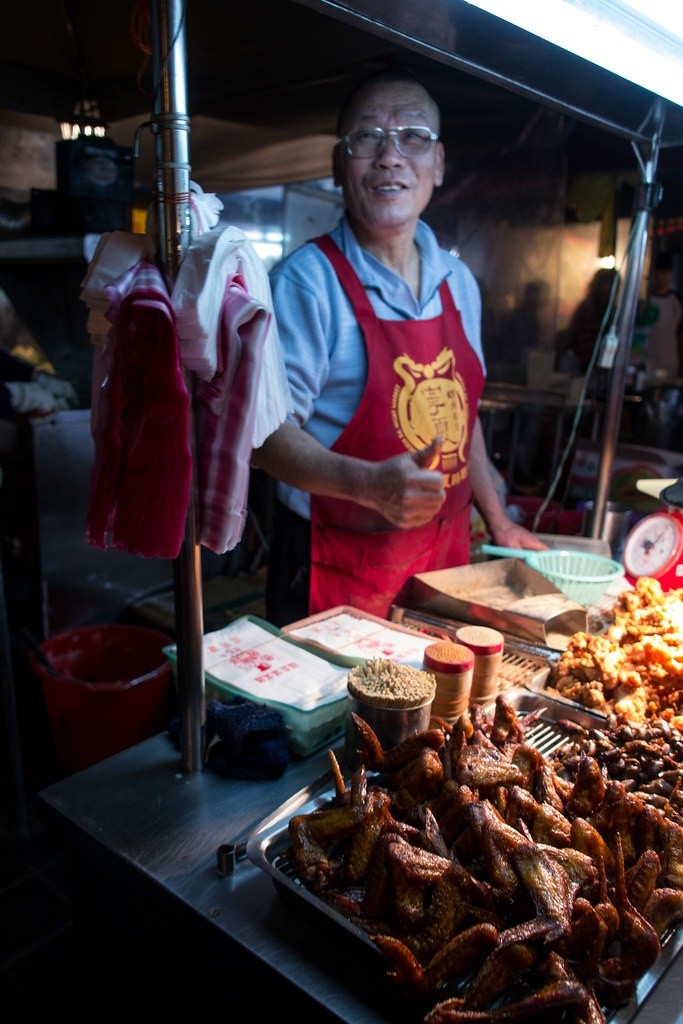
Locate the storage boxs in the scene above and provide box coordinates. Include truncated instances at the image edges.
[1,110,62,190]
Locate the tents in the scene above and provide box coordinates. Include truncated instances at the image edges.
[135,1,683,784]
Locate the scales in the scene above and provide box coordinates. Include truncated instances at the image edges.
[619,475,683,595]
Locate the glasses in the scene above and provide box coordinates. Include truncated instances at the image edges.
[338,128,440,160]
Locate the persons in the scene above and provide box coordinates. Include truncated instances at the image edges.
[570,251,683,395]
[256,69,550,620]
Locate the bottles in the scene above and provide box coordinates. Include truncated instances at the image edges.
[632,362,647,394]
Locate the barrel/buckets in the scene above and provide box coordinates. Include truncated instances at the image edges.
[504,495,559,534]
[555,508,585,536]
[31,624,176,770]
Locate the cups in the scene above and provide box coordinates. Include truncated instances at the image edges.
[584,499,632,556]
